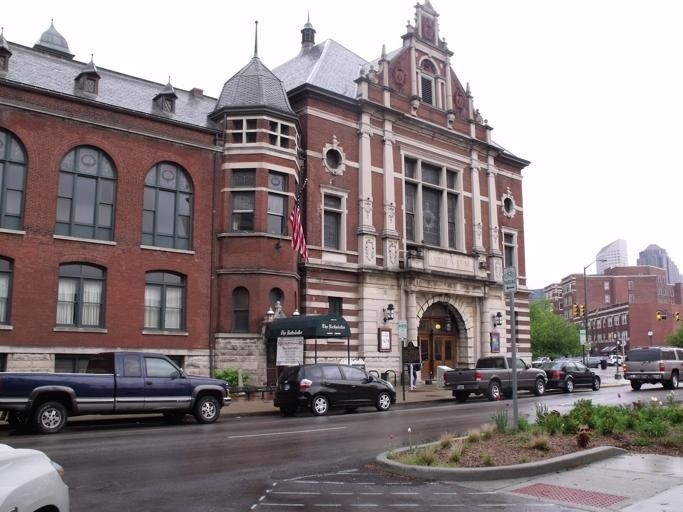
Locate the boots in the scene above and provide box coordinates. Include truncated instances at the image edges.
[621,347,682,391]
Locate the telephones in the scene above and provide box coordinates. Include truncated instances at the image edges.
[502,266,516,293]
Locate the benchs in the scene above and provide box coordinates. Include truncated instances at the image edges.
[289,192,309,264]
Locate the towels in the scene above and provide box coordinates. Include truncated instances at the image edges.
[600,360,606,370]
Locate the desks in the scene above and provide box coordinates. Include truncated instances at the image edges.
[613,340,621,380]
[582,259,607,343]
[646,329,653,346]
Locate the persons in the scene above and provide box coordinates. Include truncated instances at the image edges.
[407,364,417,389]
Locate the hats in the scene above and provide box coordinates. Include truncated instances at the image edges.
[271,357,399,417]
[537,359,602,394]
[0,440,74,512]
[530,355,626,369]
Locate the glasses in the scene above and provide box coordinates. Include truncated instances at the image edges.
[491,311,502,327]
[382,304,394,322]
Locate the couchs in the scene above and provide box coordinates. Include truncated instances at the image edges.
[571,303,579,318]
[578,303,585,317]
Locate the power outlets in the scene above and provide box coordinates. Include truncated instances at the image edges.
[442,354,549,402]
[0,351,232,436]
